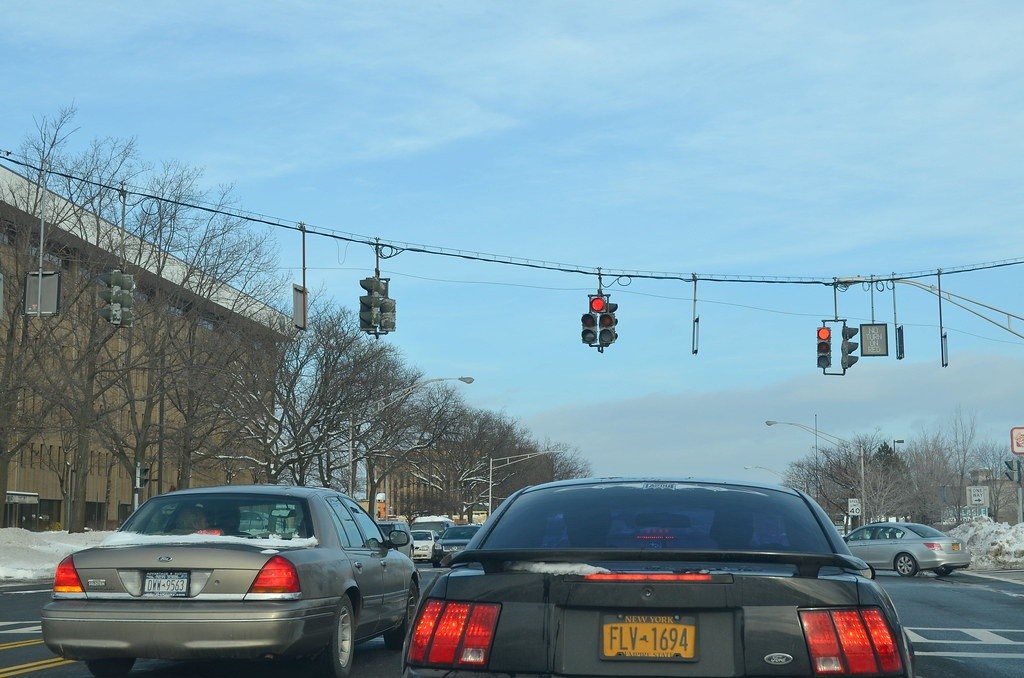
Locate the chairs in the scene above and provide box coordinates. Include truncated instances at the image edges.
[878,530,936,539]
[170,507,224,536]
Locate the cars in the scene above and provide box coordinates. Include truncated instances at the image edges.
[839,522,973,577]
[370,515,488,567]
[42,482,425,677]
[396,475,917,677]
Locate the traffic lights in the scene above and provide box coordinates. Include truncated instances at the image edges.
[581,295,622,350]
[1004,461,1019,484]
[815,328,834,371]
[840,324,863,370]
[358,275,397,339]
[100,270,138,329]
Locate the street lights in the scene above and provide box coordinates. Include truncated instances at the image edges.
[487,450,570,518]
[347,375,478,502]
[764,419,868,527]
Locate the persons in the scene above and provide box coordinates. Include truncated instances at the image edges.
[173,504,210,535]
